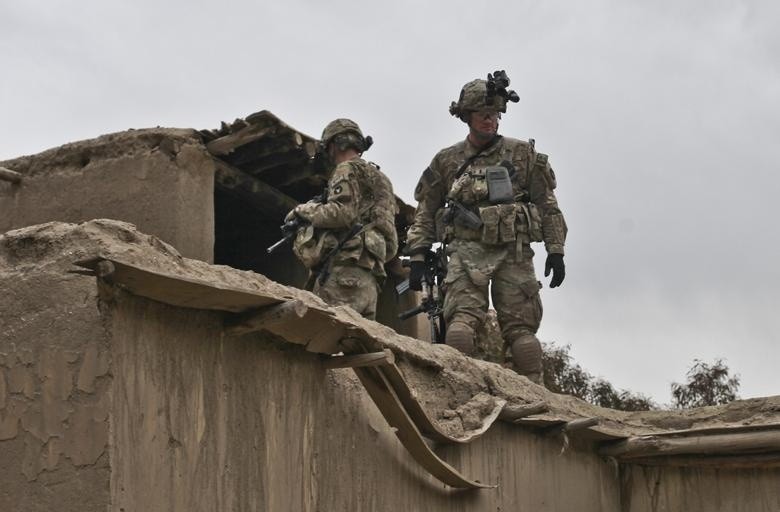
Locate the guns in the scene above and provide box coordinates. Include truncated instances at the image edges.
[265,193,326,264]
[396,243,446,343]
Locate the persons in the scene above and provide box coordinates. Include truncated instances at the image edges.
[284,118,398,324]
[406,69,566,388]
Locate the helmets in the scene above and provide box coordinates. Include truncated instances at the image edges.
[319,118,365,157]
[448,78,508,123]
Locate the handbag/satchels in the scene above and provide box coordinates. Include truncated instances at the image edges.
[292,220,340,271]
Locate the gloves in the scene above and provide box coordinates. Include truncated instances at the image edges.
[544,253,566,288]
[408,261,435,292]
